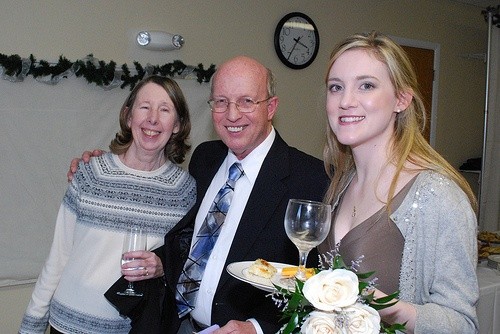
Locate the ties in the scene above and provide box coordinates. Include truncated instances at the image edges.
[175,163,245,318]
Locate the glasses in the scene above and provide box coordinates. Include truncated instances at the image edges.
[207,94,275,113]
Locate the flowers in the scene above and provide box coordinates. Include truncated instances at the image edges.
[264,242,409,334]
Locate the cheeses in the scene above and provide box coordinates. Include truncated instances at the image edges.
[282,267,315,279]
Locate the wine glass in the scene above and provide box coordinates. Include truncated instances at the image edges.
[116,227,148,296]
[279,199,331,291]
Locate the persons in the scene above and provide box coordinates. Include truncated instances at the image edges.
[315,30,480,334]
[66,54,349,334]
[17,75,197,334]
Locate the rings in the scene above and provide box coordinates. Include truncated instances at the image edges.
[145,270,149,276]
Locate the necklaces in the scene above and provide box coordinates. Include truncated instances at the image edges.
[351,160,395,218]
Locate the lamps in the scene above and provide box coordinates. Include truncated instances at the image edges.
[135,30,184,52]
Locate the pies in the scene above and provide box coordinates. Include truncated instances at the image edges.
[477,230,500,258]
[248,258,277,278]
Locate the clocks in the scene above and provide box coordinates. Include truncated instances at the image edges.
[273,11,320,70]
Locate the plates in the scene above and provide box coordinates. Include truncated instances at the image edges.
[226,260,307,295]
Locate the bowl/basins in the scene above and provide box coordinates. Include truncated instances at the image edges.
[487,255,500,269]
